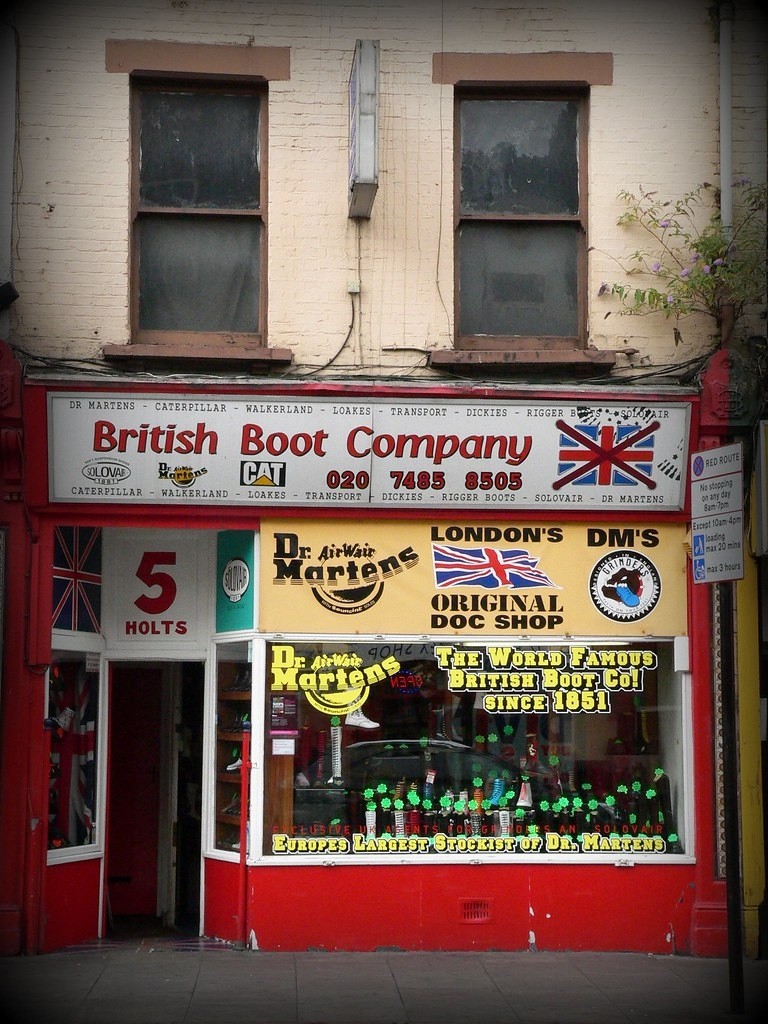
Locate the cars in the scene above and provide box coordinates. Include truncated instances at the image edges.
[292,738,624,838]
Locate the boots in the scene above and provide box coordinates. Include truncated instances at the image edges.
[184,782,201,821]
[292,673,686,858]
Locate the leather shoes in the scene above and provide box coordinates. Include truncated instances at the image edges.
[217,662,252,855]
